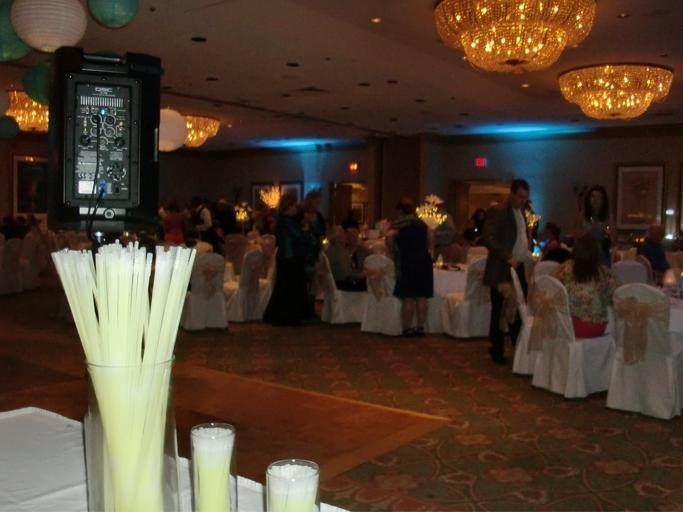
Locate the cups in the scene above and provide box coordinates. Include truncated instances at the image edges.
[80,354,181,512]
[265,457,320,512]
[189,423,238,511]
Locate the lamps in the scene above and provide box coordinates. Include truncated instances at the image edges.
[5,86,50,132]
[434,0,597,75]
[181,116,220,148]
[557,62,674,120]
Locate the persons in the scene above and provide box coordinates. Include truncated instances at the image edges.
[389,197,433,337]
[556,238,616,339]
[0,214,41,240]
[261,196,311,326]
[523,200,537,250]
[579,184,612,268]
[340,211,367,272]
[637,224,670,271]
[539,222,570,261]
[91,227,155,254]
[326,225,367,291]
[156,192,272,246]
[298,192,326,320]
[464,208,486,246]
[482,178,529,360]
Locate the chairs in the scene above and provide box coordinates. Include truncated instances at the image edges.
[223,246,264,323]
[367,239,385,255]
[179,253,228,335]
[441,255,500,338]
[314,250,367,328]
[255,234,276,280]
[533,261,559,283]
[360,255,404,337]
[635,255,657,285]
[225,234,251,282]
[0,238,23,294]
[528,274,616,400]
[606,283,683,420]
[466,247,489,263]
[511,266,539,375]
[611,260,646,283]
[19,233,41,290]
